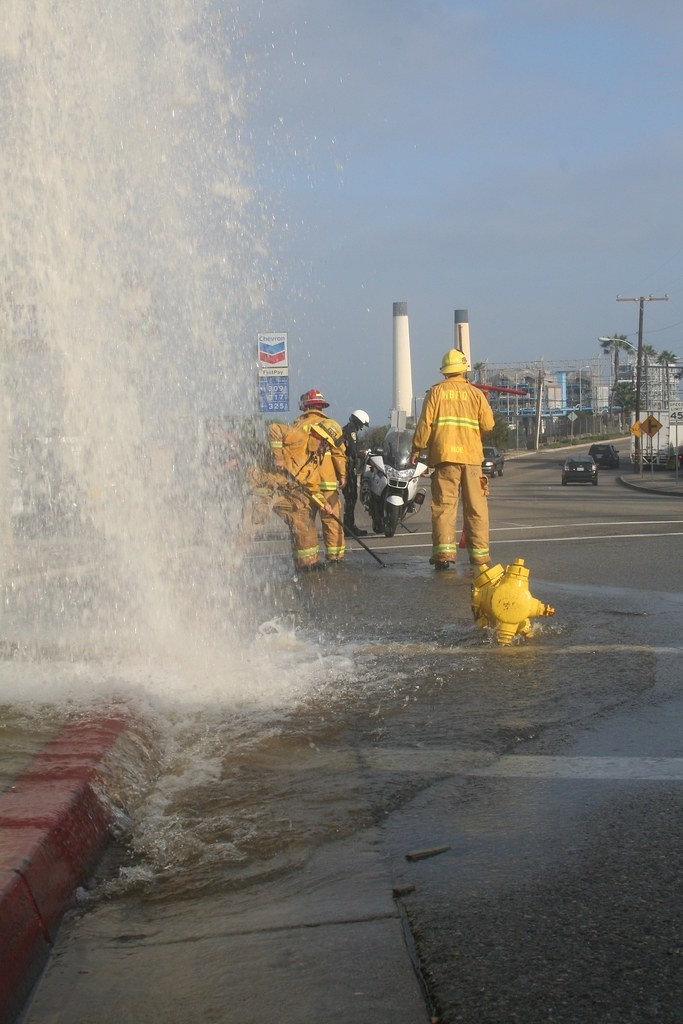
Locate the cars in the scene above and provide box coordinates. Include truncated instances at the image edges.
[481,447,508,478]
[589,444,620,469]
[558,453,600,486]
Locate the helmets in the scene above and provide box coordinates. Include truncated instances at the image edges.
[299,390,330,410]
[348,410,370,429]
[440,349,467,373]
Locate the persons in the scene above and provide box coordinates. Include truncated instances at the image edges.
[410,348,496,566]
[201,389,348,574]
[343,409,371,536]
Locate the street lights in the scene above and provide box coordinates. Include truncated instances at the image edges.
[596,336,651,462]
[578,365,590,440]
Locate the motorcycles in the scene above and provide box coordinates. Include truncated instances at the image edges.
[353,425,428,538]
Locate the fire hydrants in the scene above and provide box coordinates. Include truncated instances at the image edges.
[469,557,557,650]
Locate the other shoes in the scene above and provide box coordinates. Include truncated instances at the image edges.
[435,561,450,570]
[328,559,344,568]
[344,527,367,538]
[295,561,329,572]
